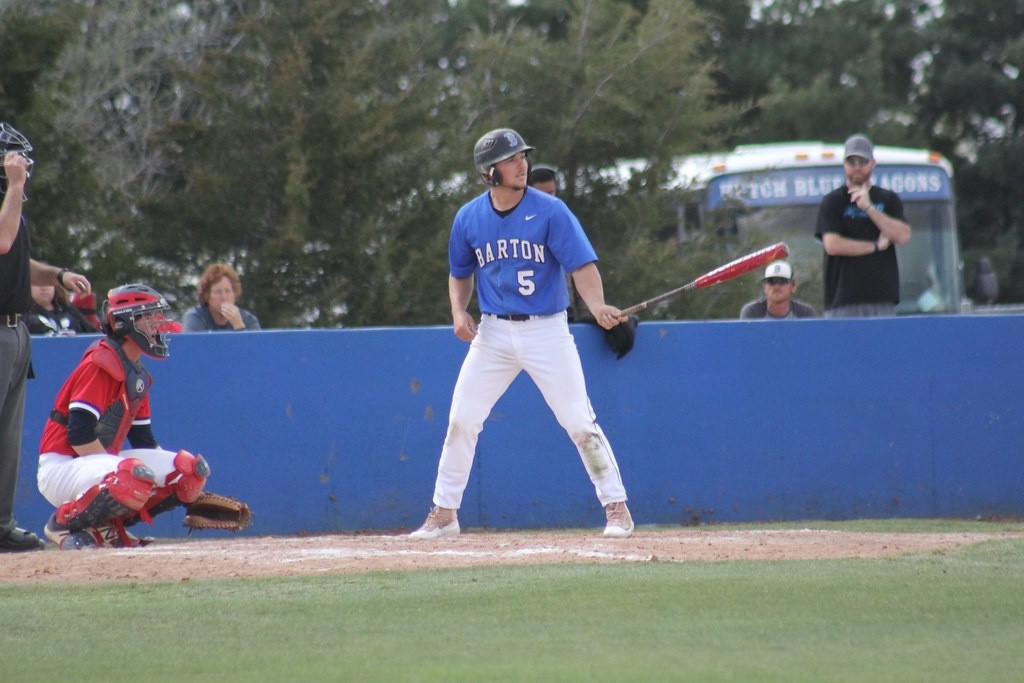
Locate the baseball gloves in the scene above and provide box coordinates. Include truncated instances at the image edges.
[181,490,256,537]
[578,312,640,361]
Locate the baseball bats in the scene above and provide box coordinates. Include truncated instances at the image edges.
[612,242,791,319]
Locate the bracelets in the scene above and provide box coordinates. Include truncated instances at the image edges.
[56,268,72,293]
[864,205,872,213]
[874,241,879,252]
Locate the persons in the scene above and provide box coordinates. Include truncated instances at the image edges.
[528,168,576,323]
[25,286,97,334]
[814,133,912,319]
[35,282,212,550]
[181,263,261,332]
[739,259,814,320]
[405,123,639,543]
[0,122,91,555]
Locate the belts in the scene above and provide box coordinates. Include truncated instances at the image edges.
[488,313,530,321]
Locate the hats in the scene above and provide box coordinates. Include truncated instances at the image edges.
[765,259,792,279]
[843,135,873,160]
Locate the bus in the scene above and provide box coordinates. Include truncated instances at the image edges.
[403,139,1000,318]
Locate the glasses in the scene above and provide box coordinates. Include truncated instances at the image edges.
[846,157,869,165]
[766,278,788,284]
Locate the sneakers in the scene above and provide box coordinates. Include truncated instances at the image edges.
[603,502,634,539]
[410,507,460,539]
[44,513,140,551]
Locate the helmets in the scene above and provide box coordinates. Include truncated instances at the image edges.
[100,283,171,360]
[474,129,536,187]
[0,121,33,203]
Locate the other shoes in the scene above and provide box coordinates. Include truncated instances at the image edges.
[0,527,45,552]
